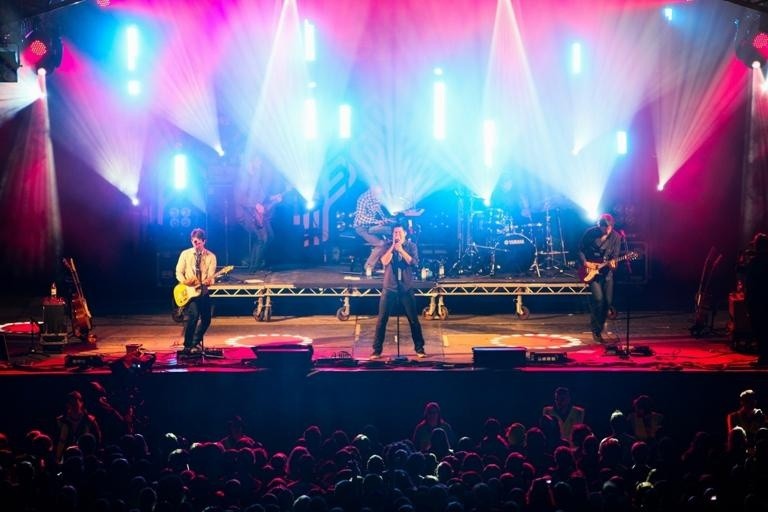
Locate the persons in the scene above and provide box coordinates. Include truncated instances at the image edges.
[578,215,622,347]
[1,381,767,512]
[173,228,218,361]
[352,178,386,280]
[370,225,430,358]
[233,157,276,277]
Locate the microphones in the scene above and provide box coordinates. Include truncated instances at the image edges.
[197,252,201,264]
[395,237,400,244]
[620,230,627,239]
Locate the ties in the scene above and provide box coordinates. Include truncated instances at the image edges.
[194,252,202,281]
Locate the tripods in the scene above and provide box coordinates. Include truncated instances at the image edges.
[449,195,577,276]
[178,268,225,362]
[700,278,727,340]
[690,272,720,332]
[14,320,51,358]
[606,248,645,359]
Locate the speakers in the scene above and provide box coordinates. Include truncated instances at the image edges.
[253,346,313,365]
[472,347,526,367]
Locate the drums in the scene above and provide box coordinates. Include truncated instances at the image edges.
[493,233,536,274]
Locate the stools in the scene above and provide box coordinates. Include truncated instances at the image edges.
[340,227,364,276]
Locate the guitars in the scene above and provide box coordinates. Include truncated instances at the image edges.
[173,265,235,308]
[59,258,92,335]
[579,250,640,284]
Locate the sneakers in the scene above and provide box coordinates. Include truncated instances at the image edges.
[370,353,381,359]
[416,352,428,358]
